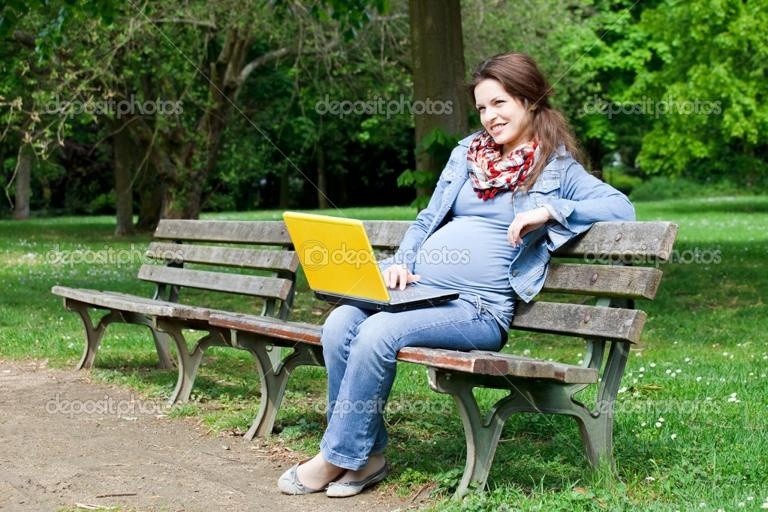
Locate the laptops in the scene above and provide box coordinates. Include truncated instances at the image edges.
[283,211,461,312]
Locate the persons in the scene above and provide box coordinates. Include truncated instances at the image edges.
[277,54,636,499]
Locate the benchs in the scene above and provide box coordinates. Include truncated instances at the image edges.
[207,219,678,499]
[48,215,299,411]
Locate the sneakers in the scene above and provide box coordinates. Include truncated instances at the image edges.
[326,462,388,498]
[277,459,323,496]
